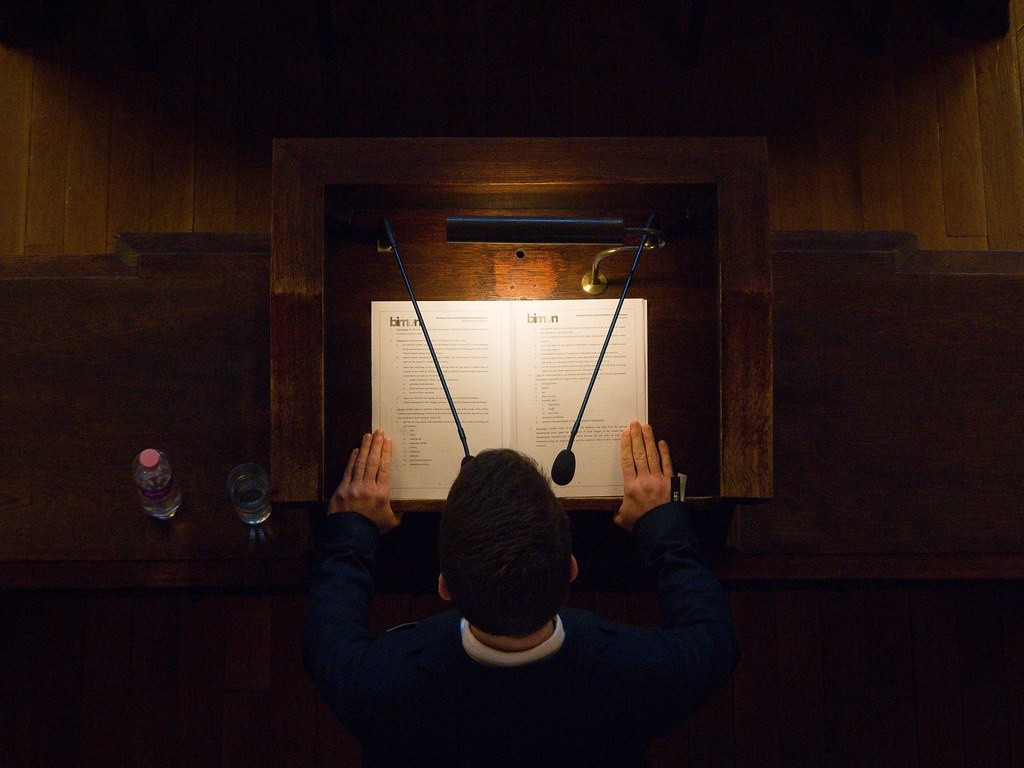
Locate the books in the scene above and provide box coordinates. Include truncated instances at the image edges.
[371,298,648,501]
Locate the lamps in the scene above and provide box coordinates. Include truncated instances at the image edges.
[444,215,667,296]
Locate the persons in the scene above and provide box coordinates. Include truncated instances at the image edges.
[305,420,738,768]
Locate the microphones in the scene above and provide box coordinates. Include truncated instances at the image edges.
[551,212,661,485]
[377,215,476,466]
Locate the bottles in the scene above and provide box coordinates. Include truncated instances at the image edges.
[132,448,181,521]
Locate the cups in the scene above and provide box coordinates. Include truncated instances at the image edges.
[226,463,272,525]
